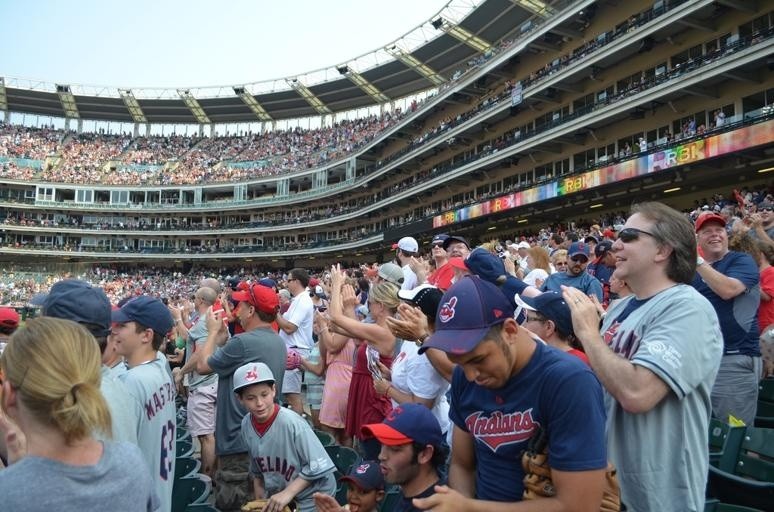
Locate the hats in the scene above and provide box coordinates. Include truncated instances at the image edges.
[448,247,508,288]
[308,277,327,299]
[566,241,591,261]
[368,262,405,286]
[397,283,446,321]
[417,273,515,358]
[392,236,419,253]
[232,361,277,395]
[227,275,291,316]
[0,307,20,328]
[514,290,572,336]
[28,279,113,339]
[337,460,385,492]
[508,241,532,250]
[694,213,728,233]
[358,402,442,451]
[109,296,175,337]
[431,234,473,251]
[584,234,617,266]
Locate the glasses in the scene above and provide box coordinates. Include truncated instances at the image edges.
[757,207,773,213]
[616,227,655,244]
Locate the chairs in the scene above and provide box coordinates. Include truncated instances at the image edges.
[325,446,359,505]
[709,418,729,467]
[754,377,774,427]
[171,396,221,512]
[312,429,336,446]
[709,426,774,483]
[705,465,774,512]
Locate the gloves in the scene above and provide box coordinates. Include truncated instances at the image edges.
[286,349,301,371]
